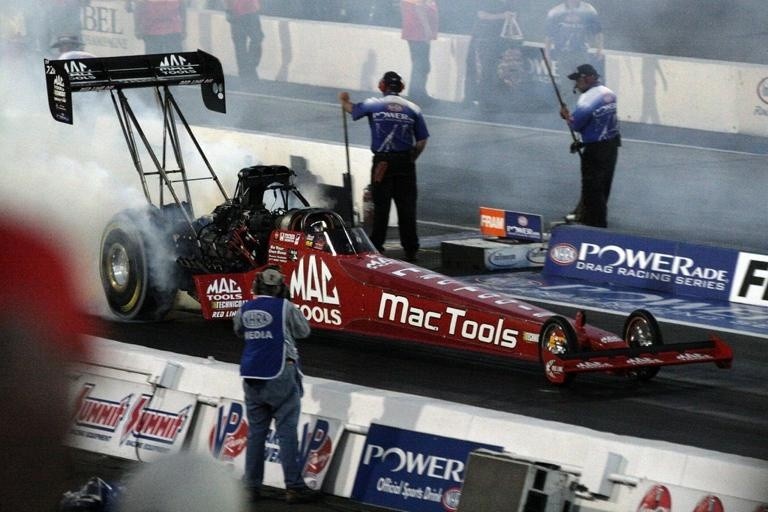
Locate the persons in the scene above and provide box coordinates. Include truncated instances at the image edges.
[50,31,85,54]
[562,65,621,228]
[545,0,605,70]
[400,0,439,106]
[475,0,519,104]
[134,0,186,54]
[340,70,429,261]
[225,0,262,81]
[233,271,321,504]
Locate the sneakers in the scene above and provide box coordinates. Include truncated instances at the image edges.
[404,253,418,263]
[283,485,325,505]
[233,483,262,503]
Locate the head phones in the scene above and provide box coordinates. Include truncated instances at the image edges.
[252,265,290,298]
[379,71,404,92]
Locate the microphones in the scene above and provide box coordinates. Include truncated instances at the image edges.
[573,84,577,94]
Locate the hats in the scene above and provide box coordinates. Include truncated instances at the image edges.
[261,267,284,286]
[566,63,598,81]
[383,71,401,89]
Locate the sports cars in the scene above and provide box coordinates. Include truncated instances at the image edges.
[42,49,739,388]
